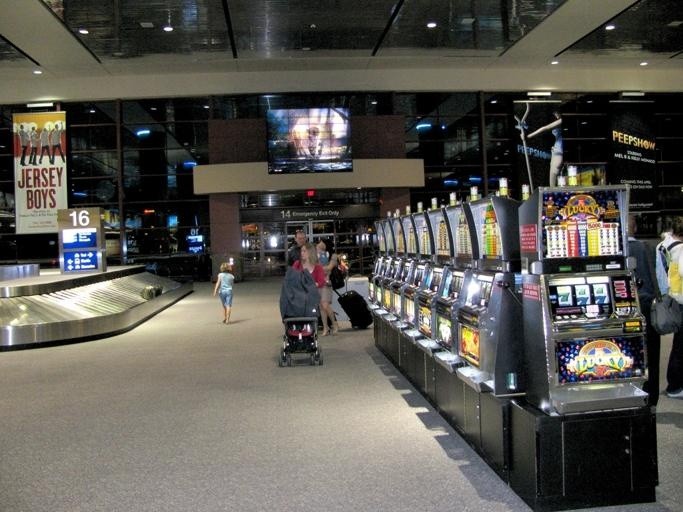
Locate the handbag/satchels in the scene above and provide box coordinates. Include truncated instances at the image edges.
[649,293,683,335]
[329,267,346,290]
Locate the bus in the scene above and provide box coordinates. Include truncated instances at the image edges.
[0,209,19,258]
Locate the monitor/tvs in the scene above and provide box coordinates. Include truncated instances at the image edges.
[548,275,615,324]
[372,257,493,313]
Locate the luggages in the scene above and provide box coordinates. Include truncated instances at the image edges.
[332,287,374,330]
[141,283,163,301]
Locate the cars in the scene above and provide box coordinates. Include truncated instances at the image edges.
[100,229,206,257]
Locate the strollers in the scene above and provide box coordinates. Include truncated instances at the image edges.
[279,268,323,370]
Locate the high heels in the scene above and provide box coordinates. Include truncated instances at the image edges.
[321,326,330,336]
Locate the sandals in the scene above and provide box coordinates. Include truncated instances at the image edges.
[331,323,339,336]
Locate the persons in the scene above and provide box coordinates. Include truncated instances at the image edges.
[293,243,324,288]
[316,239,338,336]
[287,228,309,264]
[18,124,32,166]
[213,262,235,326]
[50,123,66,164]
[26,126,40,165]
[627,214,660,415]
[38,128,51,164]
[314,241,329,267]
[656,218,682,398]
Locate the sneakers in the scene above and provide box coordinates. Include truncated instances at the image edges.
[666,387,683,399]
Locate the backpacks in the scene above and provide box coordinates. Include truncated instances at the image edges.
[654,240,683,296]
